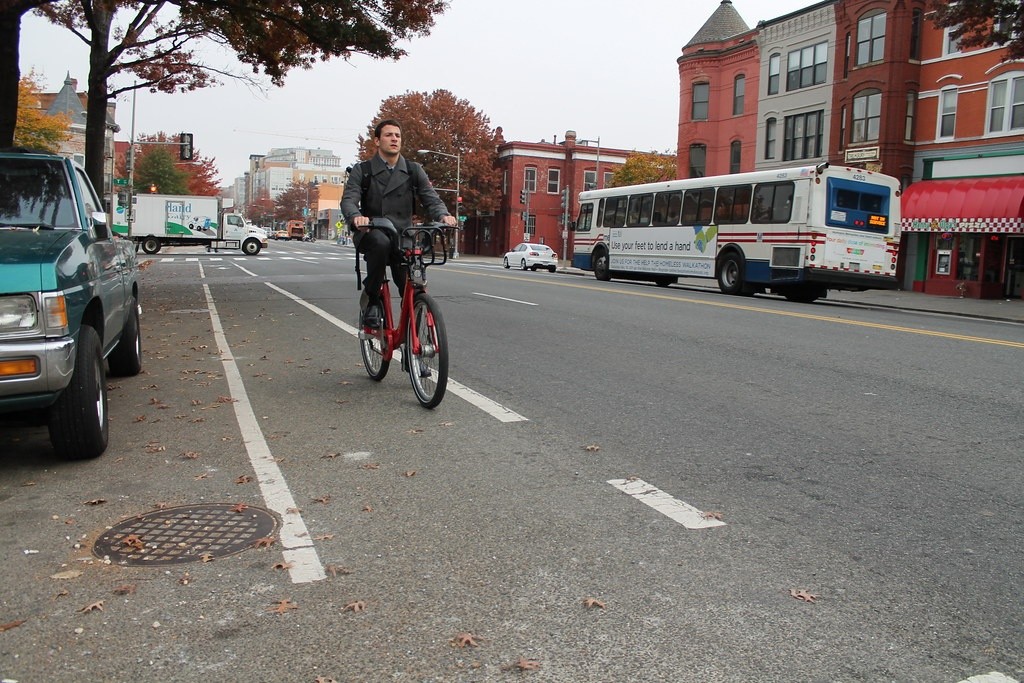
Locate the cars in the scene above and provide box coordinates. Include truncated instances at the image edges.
[261,225,290,241]
[503,242,559,273]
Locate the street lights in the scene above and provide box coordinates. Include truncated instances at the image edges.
[417,148,460,260]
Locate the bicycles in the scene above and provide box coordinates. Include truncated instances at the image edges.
[346,216,461,409]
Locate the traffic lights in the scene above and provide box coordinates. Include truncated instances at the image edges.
[150,185,157,192]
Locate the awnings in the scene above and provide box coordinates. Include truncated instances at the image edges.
[901,176,1024,234]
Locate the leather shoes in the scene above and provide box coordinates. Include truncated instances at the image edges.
[363,305,380,328]
[402,356,433,378]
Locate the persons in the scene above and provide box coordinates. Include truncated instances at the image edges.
[667,212,678,222]
[340,120,457,376]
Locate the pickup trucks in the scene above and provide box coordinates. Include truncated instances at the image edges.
[0,153,144,459]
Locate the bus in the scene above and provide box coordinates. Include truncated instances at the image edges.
[569,160,906,302]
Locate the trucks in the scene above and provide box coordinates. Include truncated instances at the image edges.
[111,192,269,257]
[288,219,305,241]
